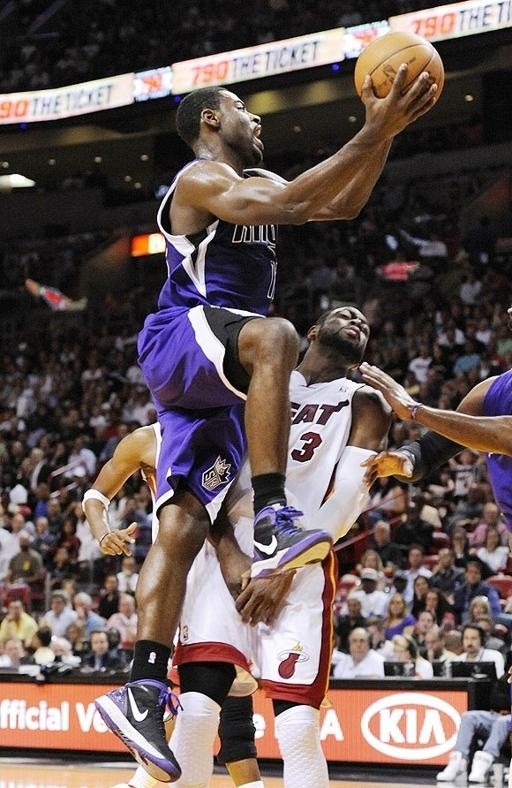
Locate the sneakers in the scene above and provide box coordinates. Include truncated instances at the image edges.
[436,751,466,784]
[94,678,184,782]
[250,502,335,579]
[468,749,492,784]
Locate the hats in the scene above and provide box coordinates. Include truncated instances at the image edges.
[360,568,377,582]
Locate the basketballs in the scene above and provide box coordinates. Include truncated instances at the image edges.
[355,30,444,110]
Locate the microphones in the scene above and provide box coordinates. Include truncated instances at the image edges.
[466,643,473,649]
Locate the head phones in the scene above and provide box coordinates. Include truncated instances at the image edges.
[89,631,113,650]
[403,633,418,658]
[346,626,374,648]
[460,624,486,646]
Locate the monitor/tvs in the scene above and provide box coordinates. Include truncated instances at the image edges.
[384,661,415,675]
[451,661,497,679]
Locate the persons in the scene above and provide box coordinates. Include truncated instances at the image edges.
[437,708,512,783]
[0,0,512,677]
[94,62,440,788]
